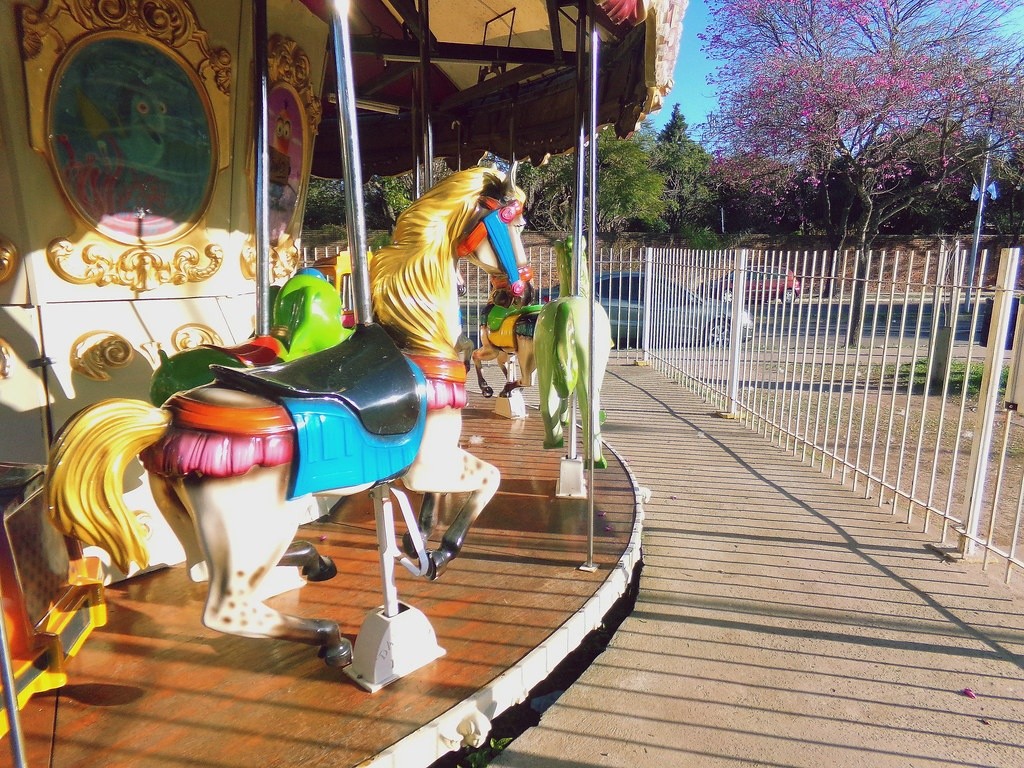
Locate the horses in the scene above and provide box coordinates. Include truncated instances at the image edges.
[41,158,614,670]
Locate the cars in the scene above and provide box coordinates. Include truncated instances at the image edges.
[534,271,753,349]
[698,264,802,305]
